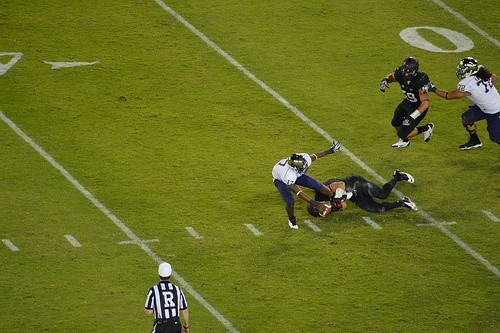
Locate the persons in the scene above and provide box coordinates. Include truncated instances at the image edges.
[272,140,340,229]
[307,168,418,217]
[144,263,190,333]
[379,57,434,147]
[428,57,500,150]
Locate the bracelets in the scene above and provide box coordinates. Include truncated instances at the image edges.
[183,325,189,329]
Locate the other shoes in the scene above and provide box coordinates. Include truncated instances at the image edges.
[333,194,345,202]
[288,220,299,229]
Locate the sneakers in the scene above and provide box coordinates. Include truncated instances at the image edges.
[392,138,410,148]
[402,197,418,211]
[459,138,482,150]
[423,122,434,142]
[393,169,414,183]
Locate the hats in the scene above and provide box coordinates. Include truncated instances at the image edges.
[158,262,172,277]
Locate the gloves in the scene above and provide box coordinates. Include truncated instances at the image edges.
[403,116,414,125]
[330,141,340,153]
[379,78,389,92]
[424,82,437,93]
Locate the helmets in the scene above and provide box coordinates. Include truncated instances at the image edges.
[288,154,307,169]
[307,202,320,217]
[455,57,478,79]
[401,57,419,80]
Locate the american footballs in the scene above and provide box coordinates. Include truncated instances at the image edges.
[319,201,332,218]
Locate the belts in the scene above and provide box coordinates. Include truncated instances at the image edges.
[157,317,178,323]
